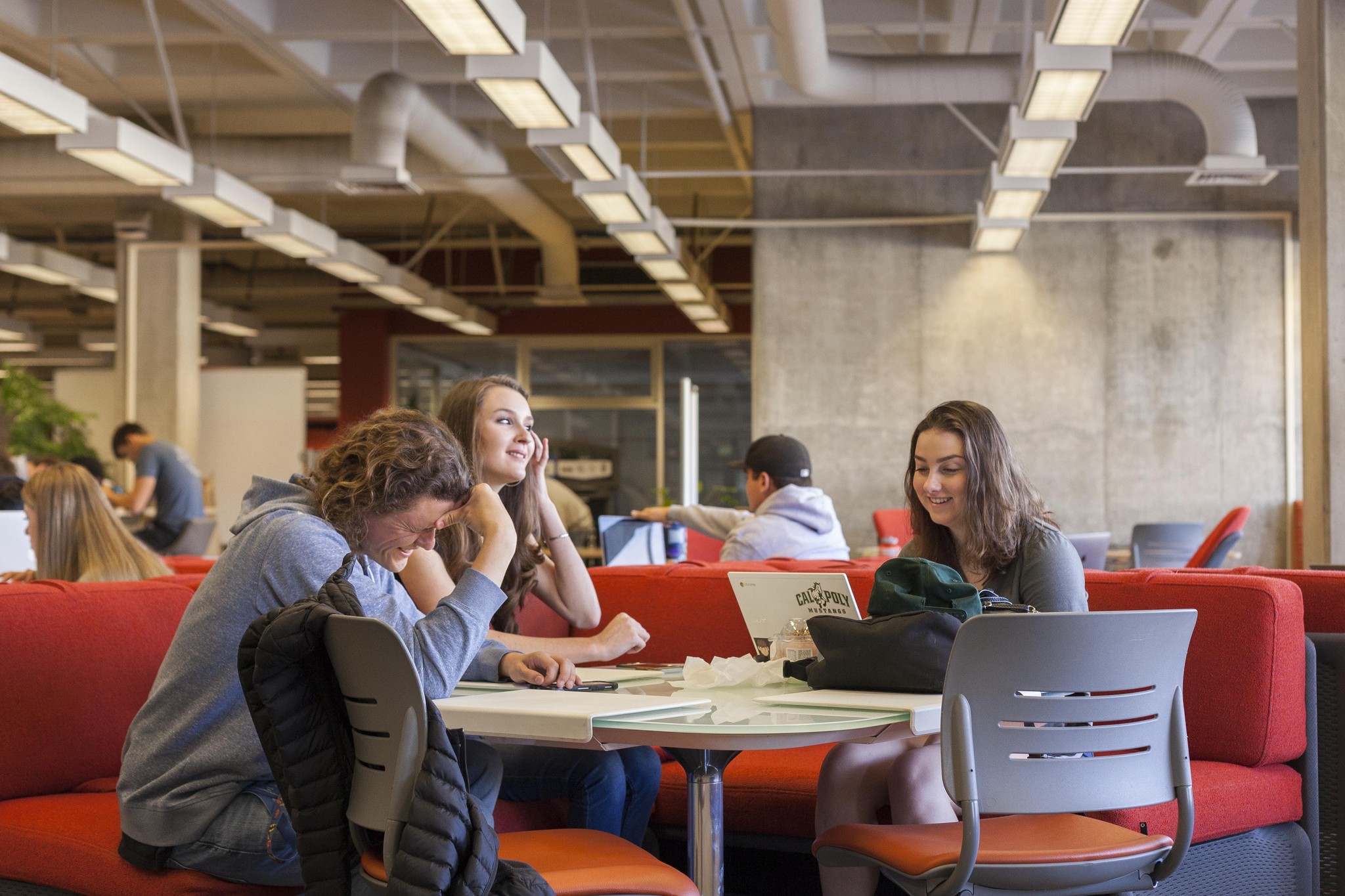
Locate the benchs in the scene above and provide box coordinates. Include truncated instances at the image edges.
[1,555,1345,896]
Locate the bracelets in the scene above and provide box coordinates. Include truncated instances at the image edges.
[544,533,569,543]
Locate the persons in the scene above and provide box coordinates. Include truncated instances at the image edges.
[391,376,663,845]
[0,461,175,584]
[111,423,205,556]
[630,434,851,563]
[116,408,580,892]
[0,450,129,511]
[812,400,1091,896]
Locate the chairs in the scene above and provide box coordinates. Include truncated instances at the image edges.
[1070,505,1252,571]
[322,614,699,896]
[811,609,1200,896]
[873,507,915,547]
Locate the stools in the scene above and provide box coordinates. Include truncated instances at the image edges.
[158,518,218,555]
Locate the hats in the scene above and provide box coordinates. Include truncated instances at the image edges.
[727,433,811,477]
[867,557,982,623]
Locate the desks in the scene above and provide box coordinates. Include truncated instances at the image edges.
[432,663,942,896]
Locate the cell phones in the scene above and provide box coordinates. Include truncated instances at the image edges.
[530,681,618,692]
[616,662,683,671]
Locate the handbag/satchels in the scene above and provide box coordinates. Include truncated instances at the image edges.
[805,611,963,693]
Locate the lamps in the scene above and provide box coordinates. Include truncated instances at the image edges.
[405,0,734,335]
[971,0,1152,252]
[1,0,499,337]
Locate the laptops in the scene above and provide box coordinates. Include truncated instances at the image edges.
[728,572,861,661]
[597,515,666,567]
